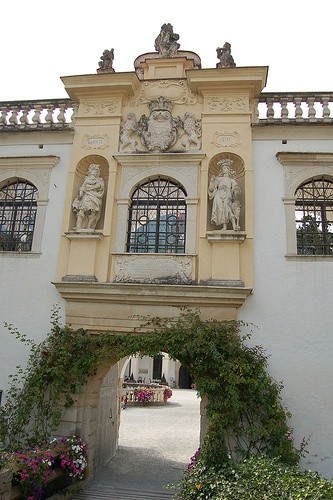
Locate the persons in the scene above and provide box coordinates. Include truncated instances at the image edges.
[207,158,242,232]
[71,163,105,232]
